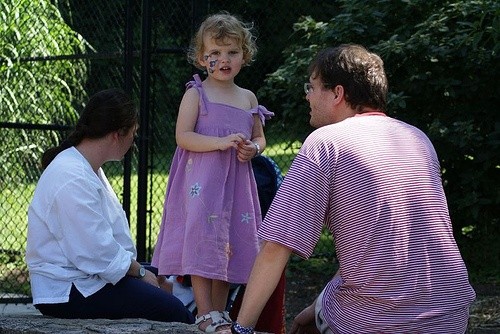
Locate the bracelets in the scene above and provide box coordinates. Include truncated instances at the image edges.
[253,143,261,158]
[231,321,254,334]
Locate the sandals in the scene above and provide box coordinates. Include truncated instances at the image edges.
[195,309,234,334]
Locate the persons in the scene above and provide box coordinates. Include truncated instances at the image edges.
[162,150,286,320]
[217,44,476,334]
[151,9,275,334]
[26,88,198,324]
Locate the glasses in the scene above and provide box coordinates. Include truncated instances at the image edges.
[304,82,323,95]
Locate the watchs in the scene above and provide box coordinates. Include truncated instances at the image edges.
[136,264,146,281]
